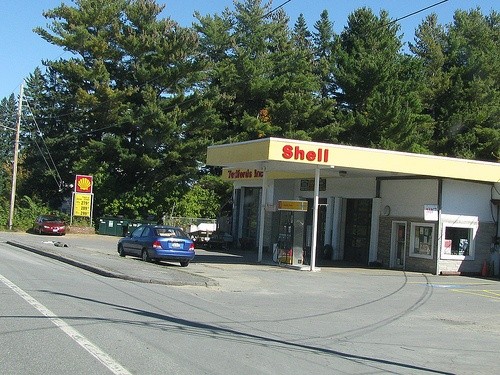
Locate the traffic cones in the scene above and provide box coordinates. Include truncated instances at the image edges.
[489,261,496,277]
[480,258,490,277]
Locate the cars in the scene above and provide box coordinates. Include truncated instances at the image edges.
[118,225,195,267]
[33,214,66,236]
